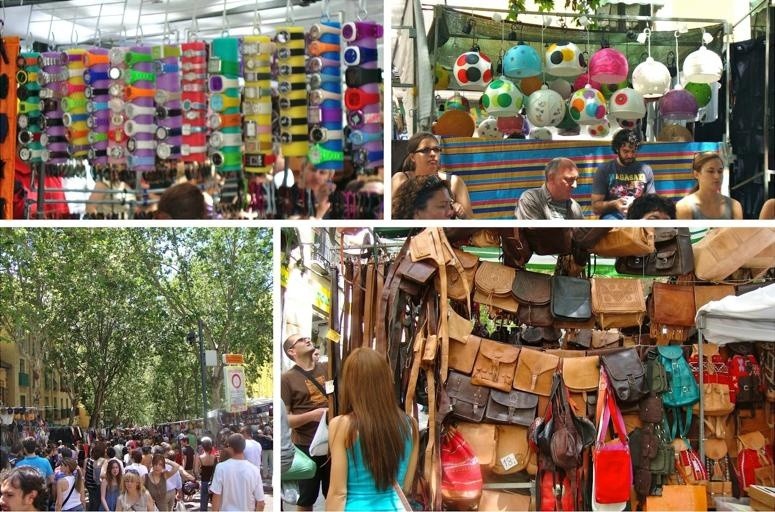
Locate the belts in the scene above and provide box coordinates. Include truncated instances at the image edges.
[332,255,437,502]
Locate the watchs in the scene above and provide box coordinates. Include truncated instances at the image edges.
[5,20,382,219]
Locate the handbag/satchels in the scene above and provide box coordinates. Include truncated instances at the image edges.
[441,227,775,512]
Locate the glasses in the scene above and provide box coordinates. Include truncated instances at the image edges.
[413,148,441,154]
[289,338,309,348]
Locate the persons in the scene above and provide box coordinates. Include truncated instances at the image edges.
[672,152,743,219]
[513,158,585,219]
[758,196,774,220]
[393,133,472,218]
[625,193,677,219]
[0,416,273,512]
[281,333,327,511]
[392,172,456,217]
[591,128,656,219]
[324,346,422,512]
